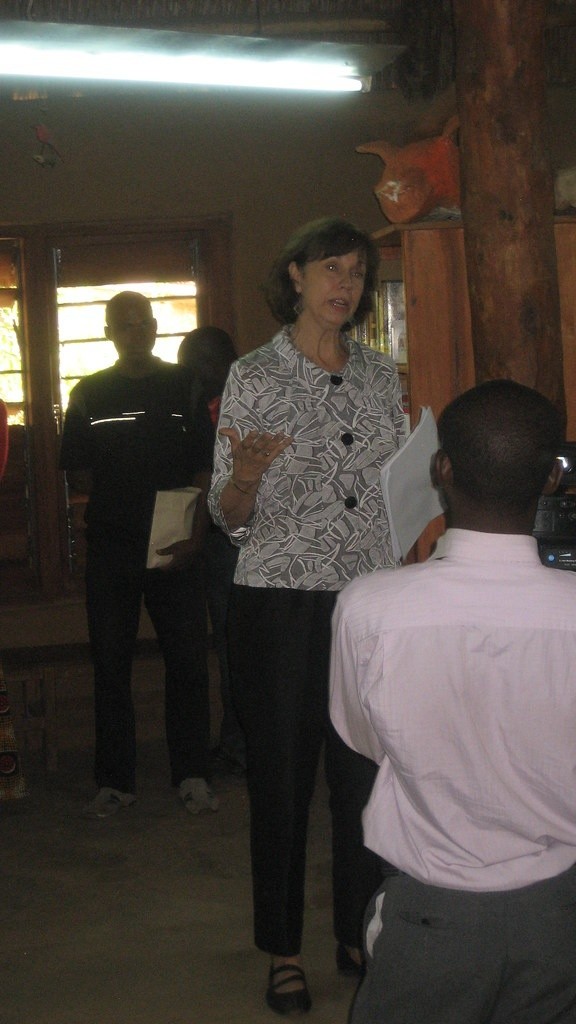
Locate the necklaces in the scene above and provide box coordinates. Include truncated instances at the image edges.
[327,379,576,1024]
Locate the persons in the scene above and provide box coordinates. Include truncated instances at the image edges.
[59,291,219,816]
[208,217,406,1018]
[182,325,246,777]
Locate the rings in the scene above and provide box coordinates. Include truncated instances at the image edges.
[264,450,270,456]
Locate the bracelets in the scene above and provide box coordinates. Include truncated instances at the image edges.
[229,478,249,494]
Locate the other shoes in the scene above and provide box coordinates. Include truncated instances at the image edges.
[335,940,369,978]
[79,786,140,821]
[265,963,313,1016]
[168,773,221,815]
